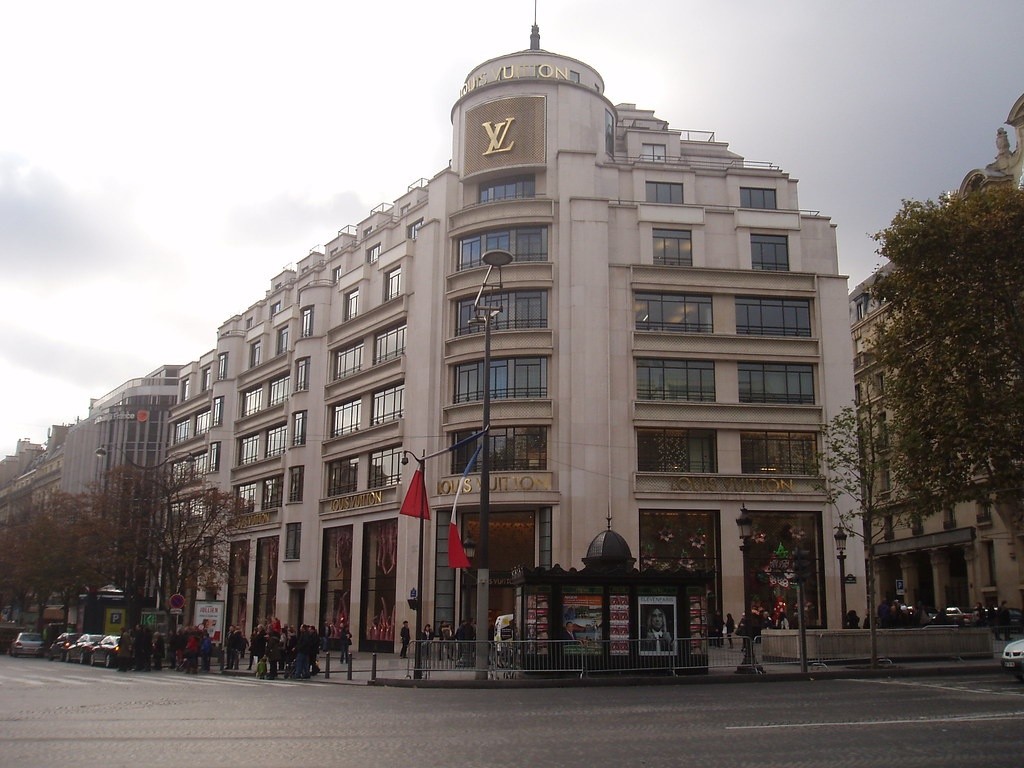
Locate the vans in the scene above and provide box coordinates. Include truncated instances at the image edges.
[947,607,962,616]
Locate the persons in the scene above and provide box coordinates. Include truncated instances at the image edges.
[562,622,577,644]
[489,618,494,665]
[735,605,786,652]
[845,599,928,629]
[643,605,673,651]
[439,618,476,661]
[246,618,320,680]
[340,627,352,664]
[713,610,734,649]
[974,601,1014,641]
[936,608,950,624]
[116,624,164,671]
[424,624,434,658]
[225,625,249,670]
[320,620,331,653]
[168,623,211,674]
[399,621,409,659]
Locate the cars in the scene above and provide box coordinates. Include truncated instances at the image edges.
[89,635,135,669]
[65,634,106,663]
[11,633,46,658]
[1001,638,1024,682]
[49,633,81,662]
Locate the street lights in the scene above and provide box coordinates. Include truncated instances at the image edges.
[834,523,847,628]
[401,449,426,676]
[735,503,765,673]
[93,443,197,626]
[468,250,515,679]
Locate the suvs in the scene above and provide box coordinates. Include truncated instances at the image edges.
[985,607,1024,631]
